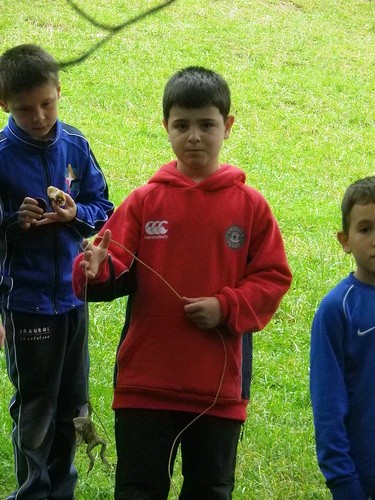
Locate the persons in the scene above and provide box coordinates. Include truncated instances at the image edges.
[0,45,115,500]
[309,175,375,500]
[73,66,292,500]
